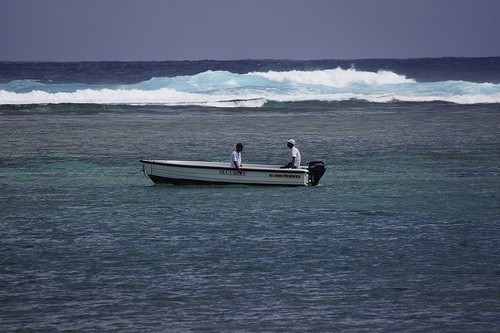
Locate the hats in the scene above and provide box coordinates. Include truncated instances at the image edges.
[236,143,245,148]
[287,139,295,145]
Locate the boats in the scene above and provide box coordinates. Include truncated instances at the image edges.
[140,160,327,188]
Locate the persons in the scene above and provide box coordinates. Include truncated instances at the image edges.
[279,138,301,169]
[230,143,245,174]
